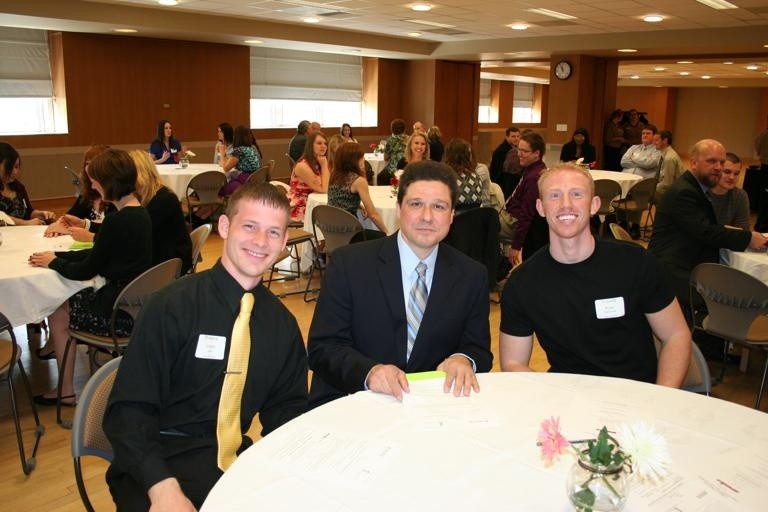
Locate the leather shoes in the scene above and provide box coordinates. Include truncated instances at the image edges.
[34,347,56,360]
[33,393,77,406]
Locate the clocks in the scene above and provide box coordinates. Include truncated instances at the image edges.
[554,61,572,80]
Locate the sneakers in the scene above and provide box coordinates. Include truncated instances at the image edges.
[630,223,640,239]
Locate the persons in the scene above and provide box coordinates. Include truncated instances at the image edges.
[26,151,155,408]
[289,118,501,303]
[0,142,55,226]
[650,140,766,318]
[490,124,684,239]
[307,161,494,416]
[649,136,766,372]
[44,143,117,238]
[213,122,234,168]
[102,179,312,512]
[250,129,264,168]
[184,125,262,231]
[707,139,767,234]
[149,120,184,164]
[60,150,196,358]
[499,162,692,389]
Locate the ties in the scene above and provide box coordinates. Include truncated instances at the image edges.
[656,156,662,181]
[216,292,255,472]
[405,262,429,363]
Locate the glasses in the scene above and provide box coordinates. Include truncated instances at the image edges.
[515,146,532,154]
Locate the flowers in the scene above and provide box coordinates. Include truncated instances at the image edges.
[171,145,196,162]
[370,141,386,152]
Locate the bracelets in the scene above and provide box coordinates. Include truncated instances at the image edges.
[83,218,91,232]
